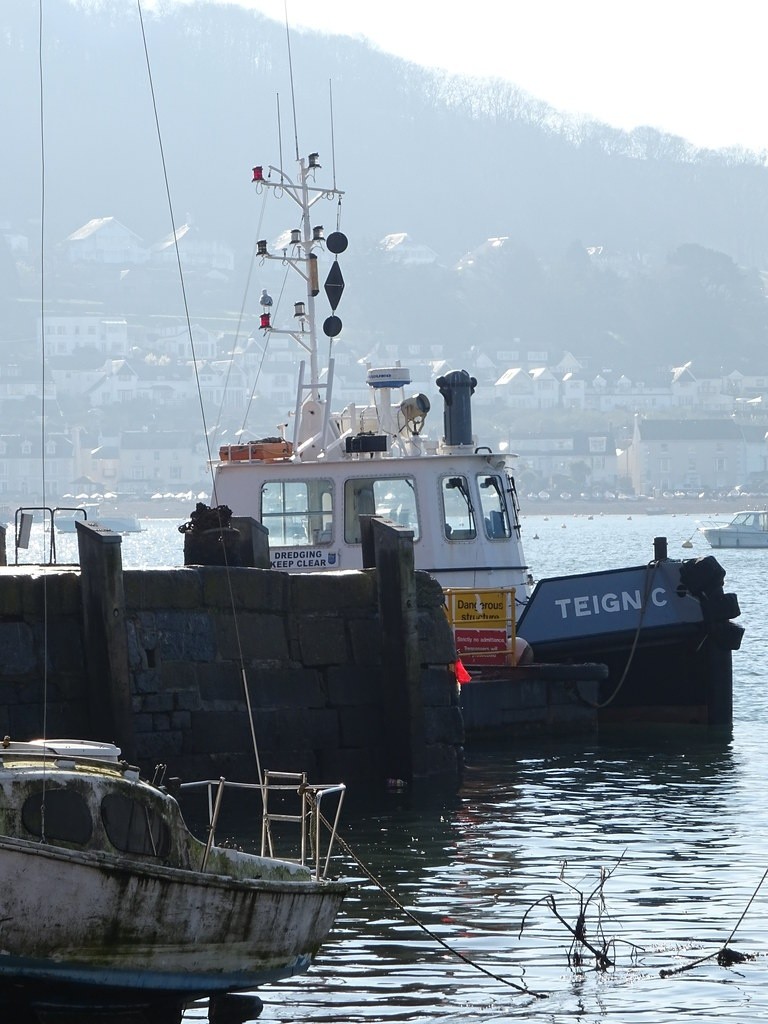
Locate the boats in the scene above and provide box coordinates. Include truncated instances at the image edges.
[54,502,142,534]
[1,723,354,995]
[176,17,532,687]
[702,510,768,551]
